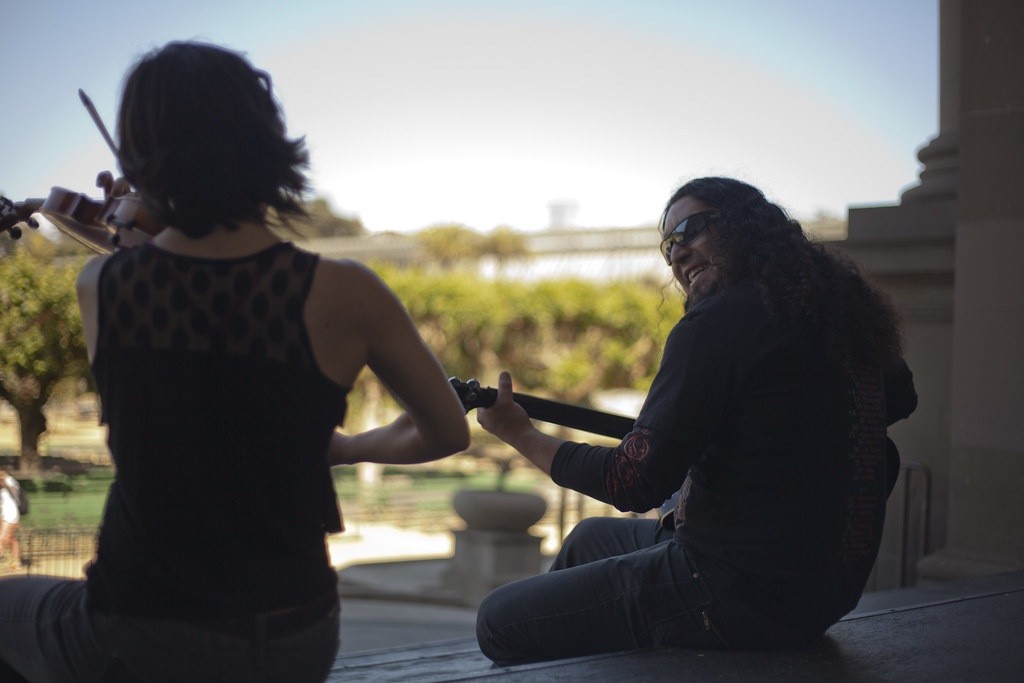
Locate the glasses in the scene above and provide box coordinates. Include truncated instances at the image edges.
[660,208,725,266]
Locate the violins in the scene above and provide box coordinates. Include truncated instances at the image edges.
[0,186,164,256]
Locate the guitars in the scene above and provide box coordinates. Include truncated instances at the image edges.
[443,375,902,538]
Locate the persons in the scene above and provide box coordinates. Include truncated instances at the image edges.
[476,176,918,665]
[1,38,471,683]
[0,468,23,570]
